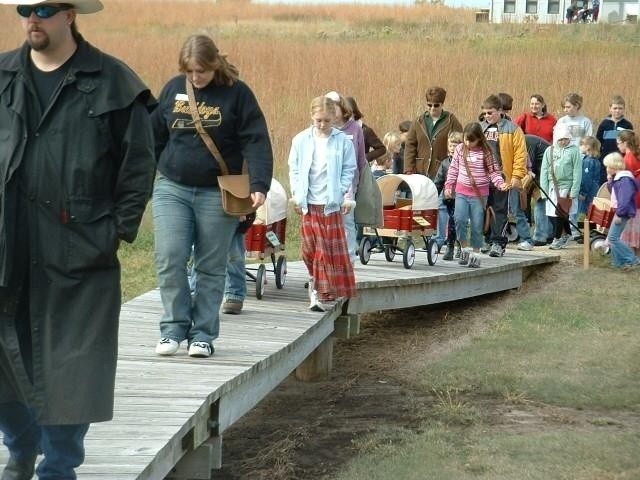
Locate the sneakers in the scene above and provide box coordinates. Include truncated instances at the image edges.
[562,233,572,248]
[489,243,503,257]
[574,235,583,240]
[469,252,480,267]
[577,239,584,245]
[443,242,454,260]
[458,251,470,265]
[222,300,242,313]
[308,292,326,311]
[188,341,211,358]
[455,242,461,258]
[156,337,179,355]
[517,240,535,250]
[548,238,563,250]
[481,241,491,253]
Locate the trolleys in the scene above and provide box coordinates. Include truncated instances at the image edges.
[532,178,613,255]
[244,178,288,300]
[359,173,439,269]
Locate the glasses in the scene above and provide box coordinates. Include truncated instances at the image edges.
[16,4,71,18]
[481,111,492,115]
[427,104,440,107]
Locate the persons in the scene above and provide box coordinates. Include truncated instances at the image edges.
[596,94,634,186]
[382,130,403,175]
[572,135,601,244]
[478,94,528,258]
[513,94,556,146]
[603,151,640,272]
[288,95,357,312]
[403,86,463,252]
[370,149,392,180]
[434,131,464,256]
[615,129,640,255]
[557,92,593,140]
[392,120,413,174]
[537,123,582,250]
[343,95,386,163]
[497,92,513,121]
[222,234,247,316]
[523,133,554,247]
[0,0,170,480]
[568,2,598,23]
[443,121,511,269]
[148,33,274,357]
[324,90,384,270]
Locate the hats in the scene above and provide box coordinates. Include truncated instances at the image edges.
[0,0,108,14]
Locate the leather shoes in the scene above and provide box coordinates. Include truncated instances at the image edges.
[0,446,43,480]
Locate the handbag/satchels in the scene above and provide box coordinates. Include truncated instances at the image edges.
[463,153,495,234]
[520,188,527,209]
[550,145,572,217]
[185,76,257,216]
